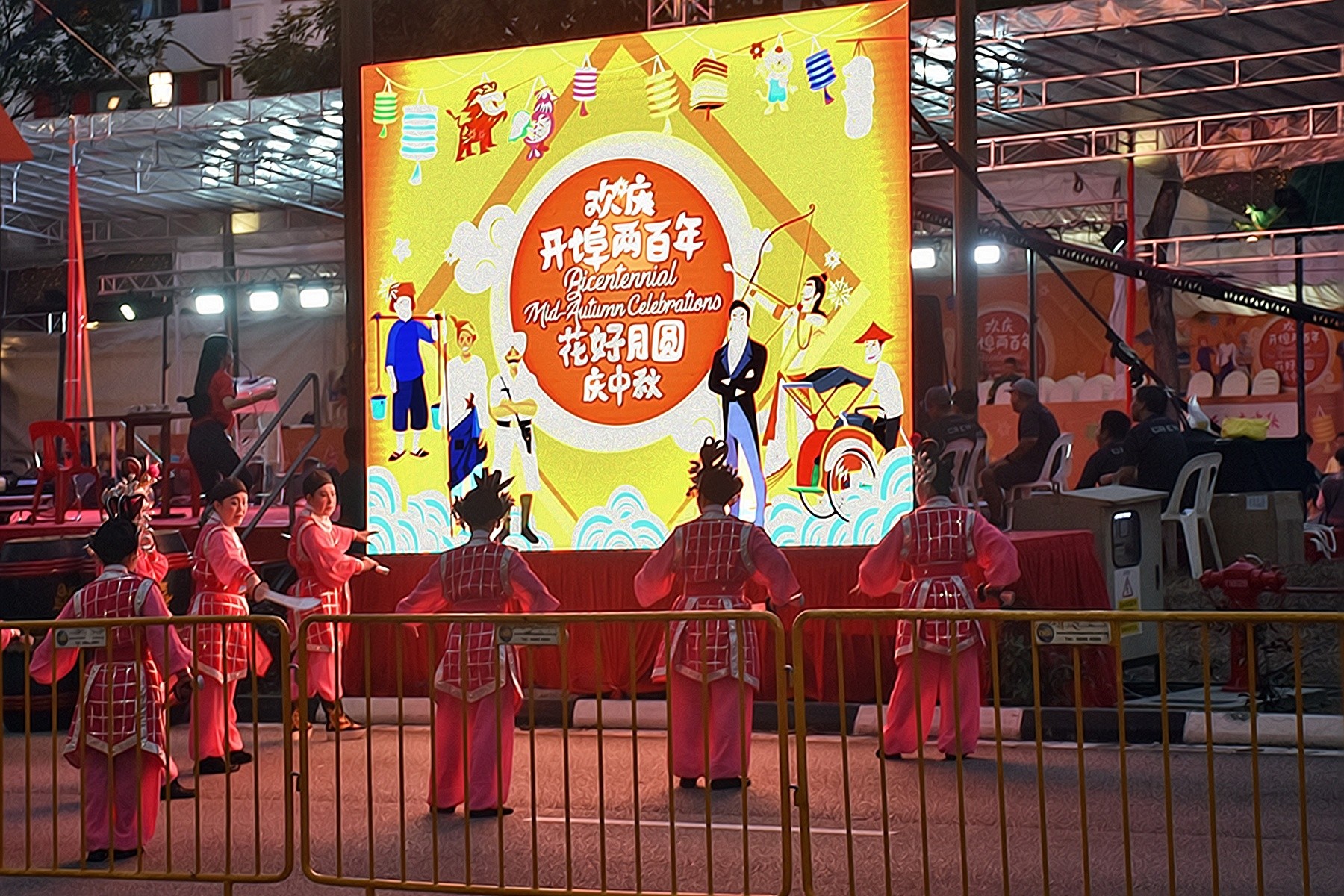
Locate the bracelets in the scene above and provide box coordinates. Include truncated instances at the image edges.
[1005,454,1016,463]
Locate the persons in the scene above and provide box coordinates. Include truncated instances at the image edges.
[27,514,195,861]
[188,334,278,503]
[984,357,1021,405]
[1071,409,1132,492]
[79,419,127,475]
[332,423,366,554]
[186,475,268,773]
[859,467,1018,760]
[980,377,1061,527]
[1095,384,1188,513]
[245,454,340,504]
[398,488,558,817]
[916,380,986,486]
[633,469,792,789]
[1302,432,1344,526]
[285,470,381,739]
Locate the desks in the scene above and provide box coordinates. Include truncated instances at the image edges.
[65,412,192,518]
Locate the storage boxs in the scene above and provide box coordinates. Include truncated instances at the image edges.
[1197,489,1306,569]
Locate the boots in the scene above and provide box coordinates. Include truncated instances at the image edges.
[319,695,367,741]
[290,702,313,741]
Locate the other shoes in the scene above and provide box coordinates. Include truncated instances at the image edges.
[429,805,456,814]
[875,749,902,760]
[193,750,251,774]
[988,516,1011,533]
[86,846,144,862]
[945,752,966,761]
[470,806,514,818]
[679,777,697,788]
[711,776,751,790]
[159,777,195,800]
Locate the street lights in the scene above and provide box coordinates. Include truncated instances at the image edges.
[148,38,242,430]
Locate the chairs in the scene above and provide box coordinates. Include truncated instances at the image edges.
[937,369,1279,579]
[29,421,104,525]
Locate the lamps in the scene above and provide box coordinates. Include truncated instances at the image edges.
[1102,225,1128,253]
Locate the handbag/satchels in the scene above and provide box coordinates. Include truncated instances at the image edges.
[1186,393,1211,433]
[1220,417,1271,440]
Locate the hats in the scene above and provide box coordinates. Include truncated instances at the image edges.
[926,387,951,410]
[302,470,334,500]
[1005,378,1037,396]
[207,471,249,502]
[92,519,139,556]
